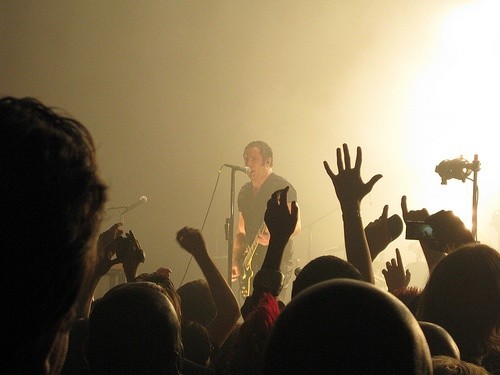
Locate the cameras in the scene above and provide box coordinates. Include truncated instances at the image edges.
[406,221,434,240]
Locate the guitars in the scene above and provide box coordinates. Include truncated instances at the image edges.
[236,196,271,302]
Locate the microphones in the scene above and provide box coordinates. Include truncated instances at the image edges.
[120,195,147,216]
[224,163,251,174]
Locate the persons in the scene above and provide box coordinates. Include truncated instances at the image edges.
[0,96,500,375]
[231,141,301,297]
